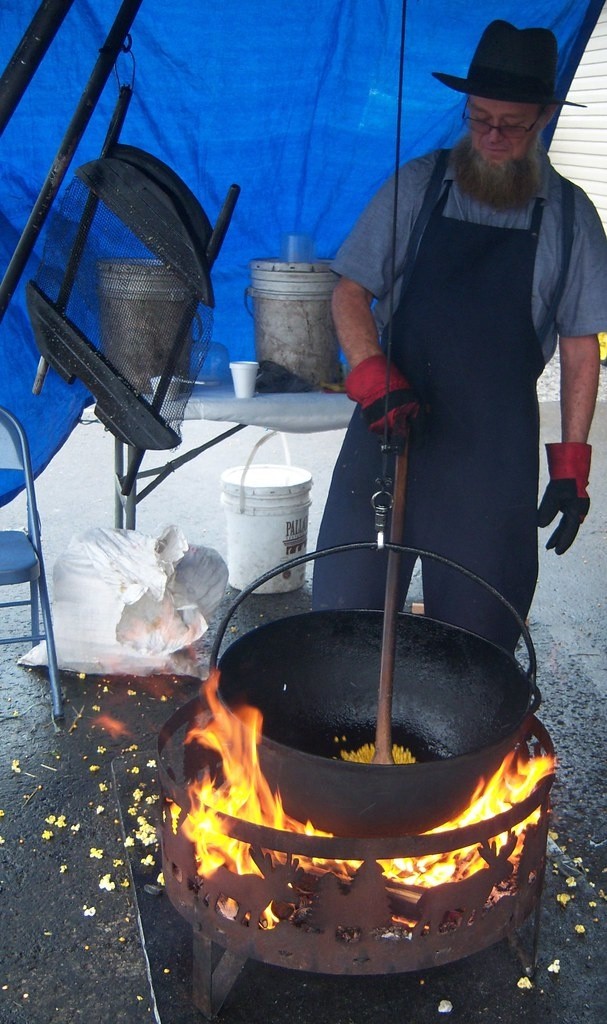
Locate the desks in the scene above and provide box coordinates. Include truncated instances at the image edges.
[79,386,369,555]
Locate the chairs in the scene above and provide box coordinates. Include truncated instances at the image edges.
[0,405,66,724]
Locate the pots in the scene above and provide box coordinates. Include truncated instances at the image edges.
[204,542,546,844]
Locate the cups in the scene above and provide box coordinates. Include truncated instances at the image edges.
[228,361,259,400]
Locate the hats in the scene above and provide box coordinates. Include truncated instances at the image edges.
[429,18,586,118]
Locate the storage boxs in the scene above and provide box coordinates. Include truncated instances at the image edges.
[94,257,191,396]
[243,256,350,392]
[221,429,315,596]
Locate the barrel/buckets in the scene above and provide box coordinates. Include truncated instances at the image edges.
[245,257,343,389]
[98,255,194,398]
[220,431,312,594]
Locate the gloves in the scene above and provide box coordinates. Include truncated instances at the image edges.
[535,439,595,558]
[345,352,423,458]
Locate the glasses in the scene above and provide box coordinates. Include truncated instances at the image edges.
[458,93,543,142]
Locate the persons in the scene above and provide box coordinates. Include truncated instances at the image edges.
[312,54,606,657]
